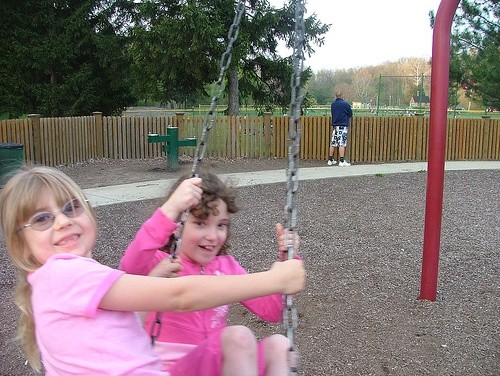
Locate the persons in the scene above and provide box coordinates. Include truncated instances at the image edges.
[0,163,306,376]
[117,167,302,376]
[327,90,352,168]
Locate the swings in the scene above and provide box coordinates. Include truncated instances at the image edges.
[148,0,306,376]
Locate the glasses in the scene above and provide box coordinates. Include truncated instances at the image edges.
[16,197,90,232]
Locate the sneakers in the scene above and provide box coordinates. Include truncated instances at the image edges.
[339,160,351,167]
[327,158,337,166]
